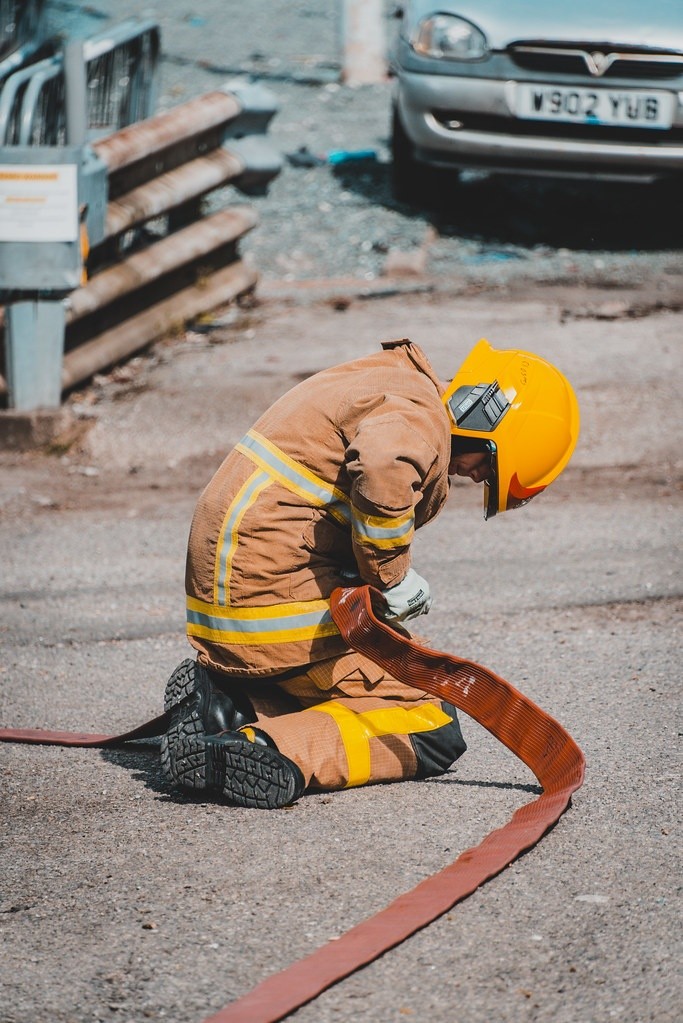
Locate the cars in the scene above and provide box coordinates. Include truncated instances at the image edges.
[383,0,683,199]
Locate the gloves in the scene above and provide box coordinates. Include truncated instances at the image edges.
[382,567,432,622]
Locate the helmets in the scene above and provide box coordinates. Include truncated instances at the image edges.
[441,337,581,521]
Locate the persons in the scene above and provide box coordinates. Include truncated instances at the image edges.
[157,338,579,807]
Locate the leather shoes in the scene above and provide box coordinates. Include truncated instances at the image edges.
[169,727,305,808]
[160,658,257,804]
